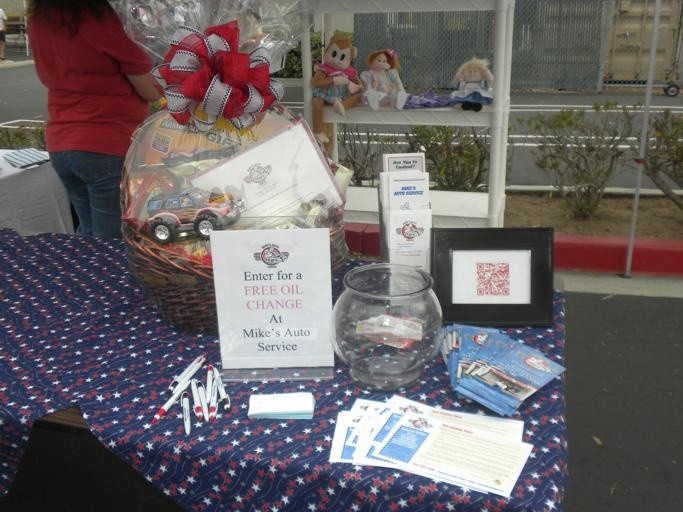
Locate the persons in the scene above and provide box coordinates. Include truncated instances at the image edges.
[448,56,493,111]
[358,48,448,107]
[23,0,163,243]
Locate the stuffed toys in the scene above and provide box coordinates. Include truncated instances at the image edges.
[312,30,362,142]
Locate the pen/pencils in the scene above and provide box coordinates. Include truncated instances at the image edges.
[151,351,231,436]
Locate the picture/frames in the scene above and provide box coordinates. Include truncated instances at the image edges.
[430,227,555,326]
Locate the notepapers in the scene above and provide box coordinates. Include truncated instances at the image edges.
[247,392,316,420]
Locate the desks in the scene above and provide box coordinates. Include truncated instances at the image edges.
[0,149,77,236]
[0,234,566,512]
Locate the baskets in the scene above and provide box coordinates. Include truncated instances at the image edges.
[120,103,350,336]
